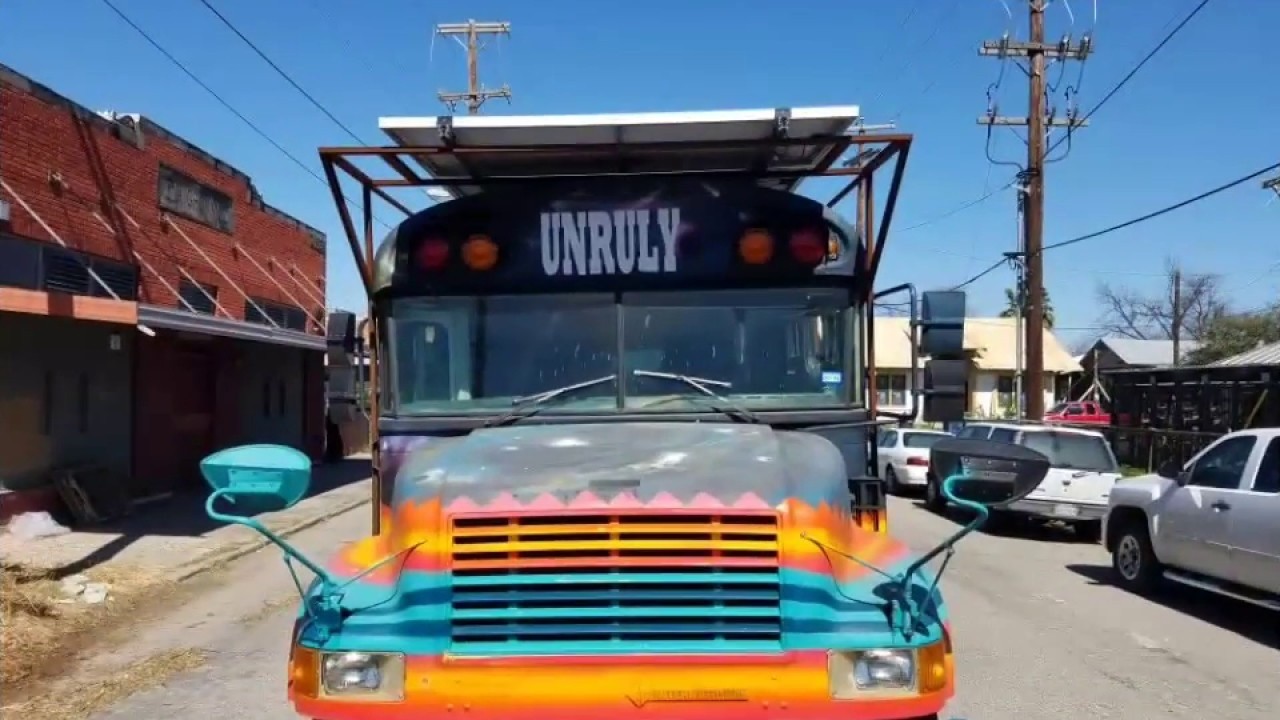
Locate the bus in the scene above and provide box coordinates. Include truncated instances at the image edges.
[202,107,1054,718]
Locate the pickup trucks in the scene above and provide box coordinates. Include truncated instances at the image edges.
[1042,400,1130,428]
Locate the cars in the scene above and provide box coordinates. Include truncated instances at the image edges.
[869,427,956,493]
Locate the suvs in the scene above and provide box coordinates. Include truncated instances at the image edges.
[1105,427,1280,615]
[926,422,1121,536]
